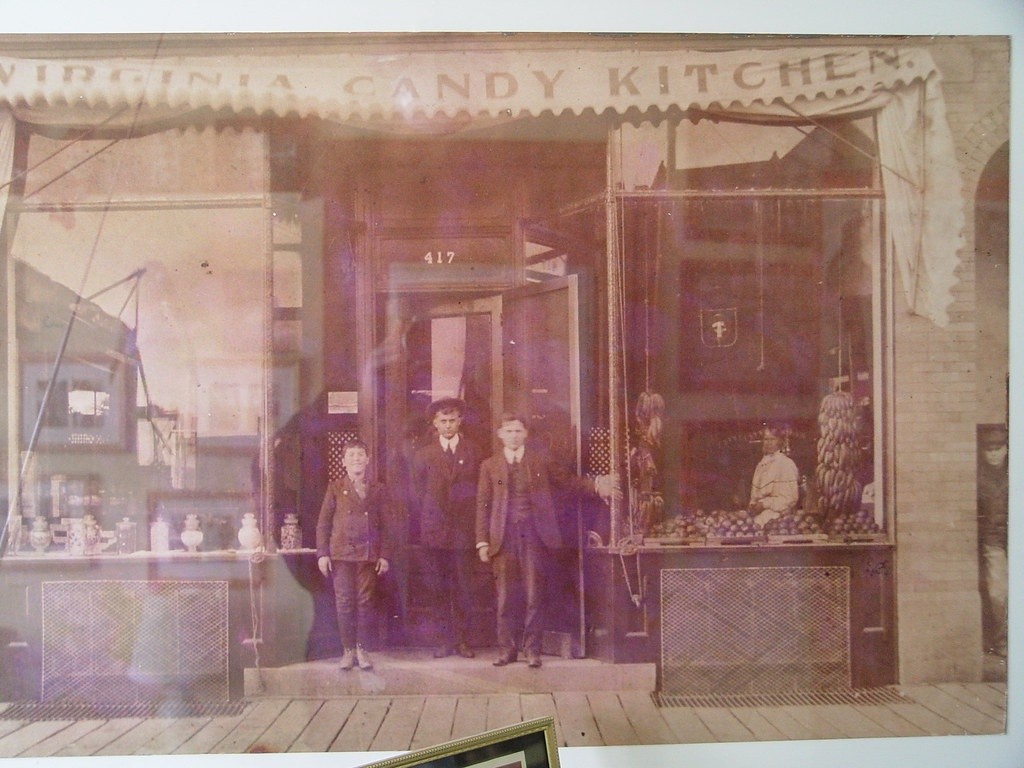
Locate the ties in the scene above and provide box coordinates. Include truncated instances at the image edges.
[445,443,455,472]
[511,456,519,468]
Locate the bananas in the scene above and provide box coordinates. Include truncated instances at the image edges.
[814,391,862,511]
[633,393,663,526]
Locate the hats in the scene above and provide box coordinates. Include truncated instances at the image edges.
[425,396,467,423]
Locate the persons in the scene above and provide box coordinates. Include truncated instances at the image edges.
[475,406,623,667]
[407,393,486,658]
[975,425,1009,660]
[745,423,801,527]
[316,440,396,670]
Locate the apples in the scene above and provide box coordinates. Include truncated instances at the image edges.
[648,507,879,538]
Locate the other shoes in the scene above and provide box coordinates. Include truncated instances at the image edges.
[527,654,541,666]
[458,644,476,658]
[340,647,372,670]
[434,645,454,657]
[494,656,517,666]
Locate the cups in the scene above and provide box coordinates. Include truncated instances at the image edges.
[115,518,136,556]
[150,517,168,551]
